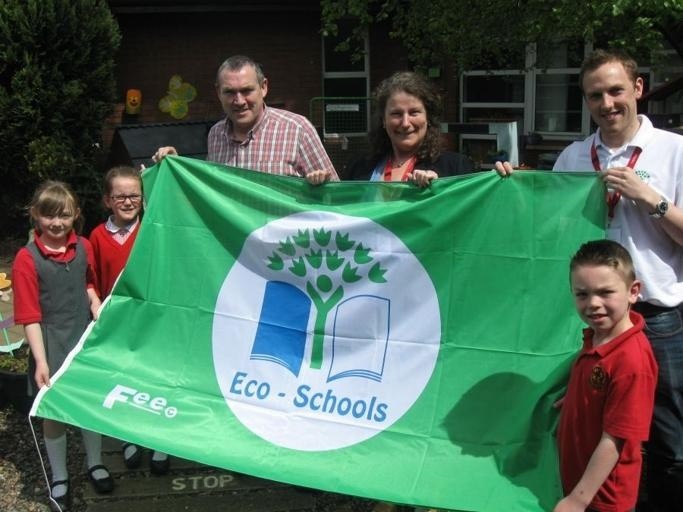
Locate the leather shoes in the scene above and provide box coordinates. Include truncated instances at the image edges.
[87,462,115,495]
[149,440,171,477]
[50,472,71,511]
[122,433,143,470]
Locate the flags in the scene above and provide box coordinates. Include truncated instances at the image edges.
[29,157,609,512]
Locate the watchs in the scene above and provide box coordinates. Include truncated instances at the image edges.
[648,195,668,221]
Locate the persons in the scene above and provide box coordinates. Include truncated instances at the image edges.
[151,55,341,186]
[89,165,170,475]
[12,181,114,512]
[346,72,475,189]
[551,240,660,512]
[494,47,683,512]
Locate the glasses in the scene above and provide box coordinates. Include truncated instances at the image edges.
[108,195,144,202]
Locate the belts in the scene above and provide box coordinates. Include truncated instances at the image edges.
[631,302,673,312]
